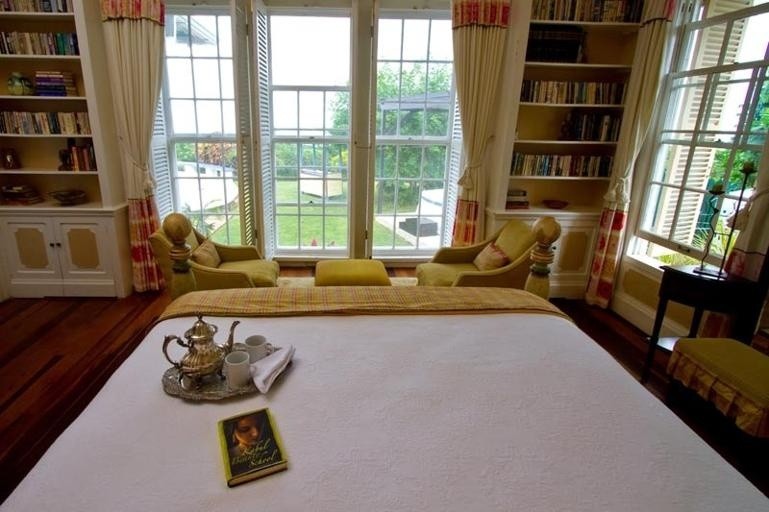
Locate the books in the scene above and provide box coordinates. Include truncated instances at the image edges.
[215,406,289,488]
[0,0,98,208]
[504,0,644,210]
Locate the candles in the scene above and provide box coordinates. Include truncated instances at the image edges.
[709,183,725,194]
[741,159,757,173]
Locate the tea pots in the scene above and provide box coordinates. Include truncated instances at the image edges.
[164,314,240,385]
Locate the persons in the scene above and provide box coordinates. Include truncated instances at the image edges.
[226,415,269,456]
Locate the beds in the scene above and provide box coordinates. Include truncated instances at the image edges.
[1,285,768,510]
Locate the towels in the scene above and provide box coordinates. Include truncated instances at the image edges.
[250,340,296,395]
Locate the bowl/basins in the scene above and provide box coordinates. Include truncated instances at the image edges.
[543,199,571,211]
[45,188,85,206]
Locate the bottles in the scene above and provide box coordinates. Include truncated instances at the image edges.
[0,148,15,169]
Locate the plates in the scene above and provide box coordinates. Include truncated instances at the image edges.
[163,347,288,404]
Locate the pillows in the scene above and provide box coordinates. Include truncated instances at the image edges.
[191,236,222,271]
[473,242,510,273]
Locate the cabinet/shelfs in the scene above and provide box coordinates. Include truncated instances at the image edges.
[1,201,137,303]
[1,1,135,211]
[483,205,606,303]
[482,1,650,212]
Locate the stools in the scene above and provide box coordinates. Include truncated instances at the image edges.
[666,334,767,444]
[315,256,393,285]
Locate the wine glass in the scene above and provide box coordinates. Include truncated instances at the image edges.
[57,148,74,171]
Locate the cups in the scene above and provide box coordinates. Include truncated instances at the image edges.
[222,334,274,388]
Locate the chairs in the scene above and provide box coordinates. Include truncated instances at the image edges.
[417,217,541,292]
[148,220,280,295]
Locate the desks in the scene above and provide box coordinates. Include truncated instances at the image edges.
[642,263,767,399]
